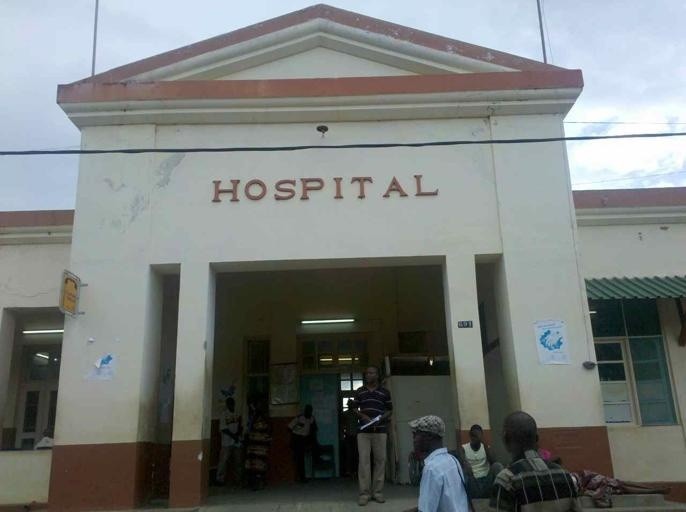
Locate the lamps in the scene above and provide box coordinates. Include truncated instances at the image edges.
[299,318,356,324]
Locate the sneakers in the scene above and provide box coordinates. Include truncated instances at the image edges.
[373,492,385,503]
[359,494,371,505]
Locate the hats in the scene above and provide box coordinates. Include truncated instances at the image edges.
[408,415,445,437]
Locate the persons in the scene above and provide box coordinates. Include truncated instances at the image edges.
[567,469,675,499]
[489,410,580,512]
[404,414,472,511]
[535,434,552,464]
[285,403,322,485]
[242,396,279,490]
[212,396,241,488]
[338,397,358,476]
[350,365,393,508]
[458,423,503,509]
